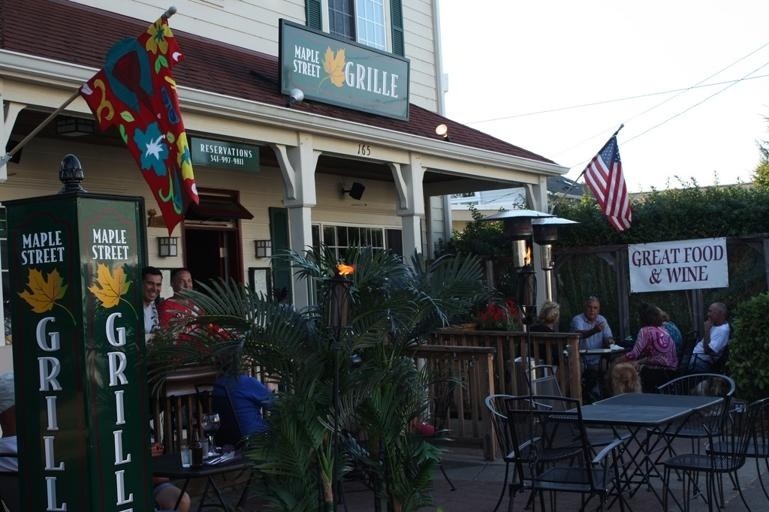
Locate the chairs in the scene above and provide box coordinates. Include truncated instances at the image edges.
[524,363,567,423]
[659,399,767,511]
[486,392,556,511]
[655,374,736,483]
[0,381,455,512]
[504,392,611,512]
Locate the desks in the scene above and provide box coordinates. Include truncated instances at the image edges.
[558,392,725,511]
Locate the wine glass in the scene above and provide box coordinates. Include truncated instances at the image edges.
[200,414,223,456]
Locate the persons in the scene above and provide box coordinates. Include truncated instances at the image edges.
[527,293,731,411]
[211,351,285,459]
[150,440,191,512]
[0,369,22,511]
[158,267,236,363]
[140,265,169,352]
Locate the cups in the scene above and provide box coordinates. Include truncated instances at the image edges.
[180,445,193,468]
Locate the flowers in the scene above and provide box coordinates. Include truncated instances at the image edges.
[477,298,526,329]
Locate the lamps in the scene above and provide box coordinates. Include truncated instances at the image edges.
[157,236,178,256]
[286,87,304,108]
[256,239,272,259]
[435,123,448,140]
[343,182,365,200]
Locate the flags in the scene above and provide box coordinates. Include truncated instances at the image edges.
[582,132,634,234]
[76,12,201,240]
